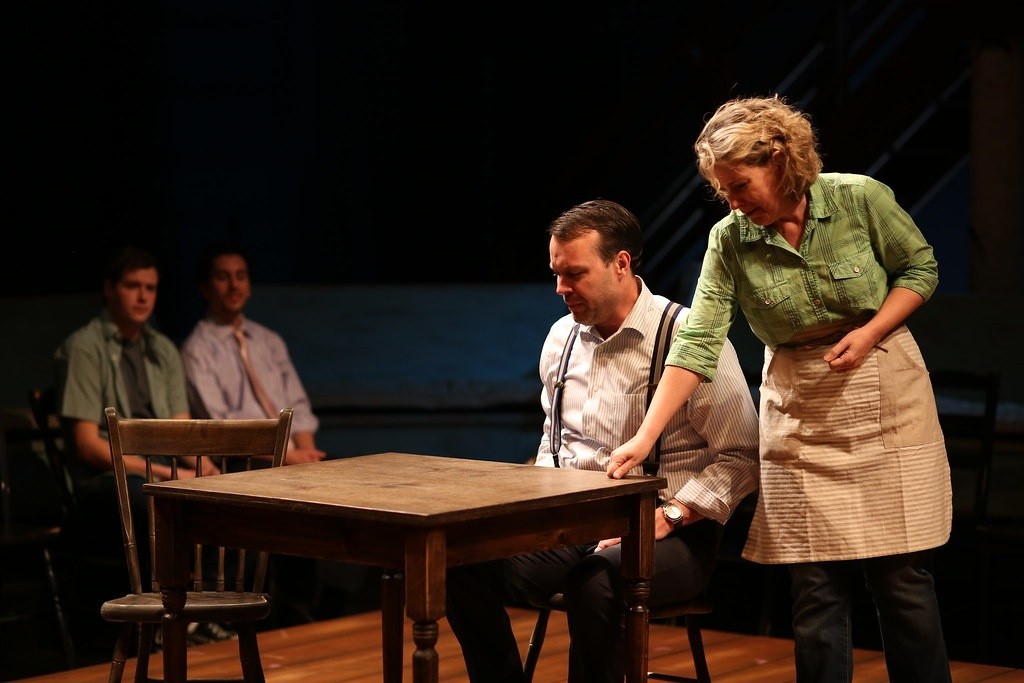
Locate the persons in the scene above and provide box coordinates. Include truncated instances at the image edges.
[605,96,953,682]
[443,199,758,683]
[60,248,239,658]
[181,246,329,633]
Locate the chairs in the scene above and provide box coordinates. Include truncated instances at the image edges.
[97,405,297,683]
[31,385,151,665]
[518,488,758,683]
[1,406,82,677]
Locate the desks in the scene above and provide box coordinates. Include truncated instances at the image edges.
[138,450,670,683]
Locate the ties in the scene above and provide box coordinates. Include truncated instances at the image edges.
[234,329,296,450]
[119,336,169,461]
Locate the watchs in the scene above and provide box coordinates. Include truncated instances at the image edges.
[659,501,682,530]
